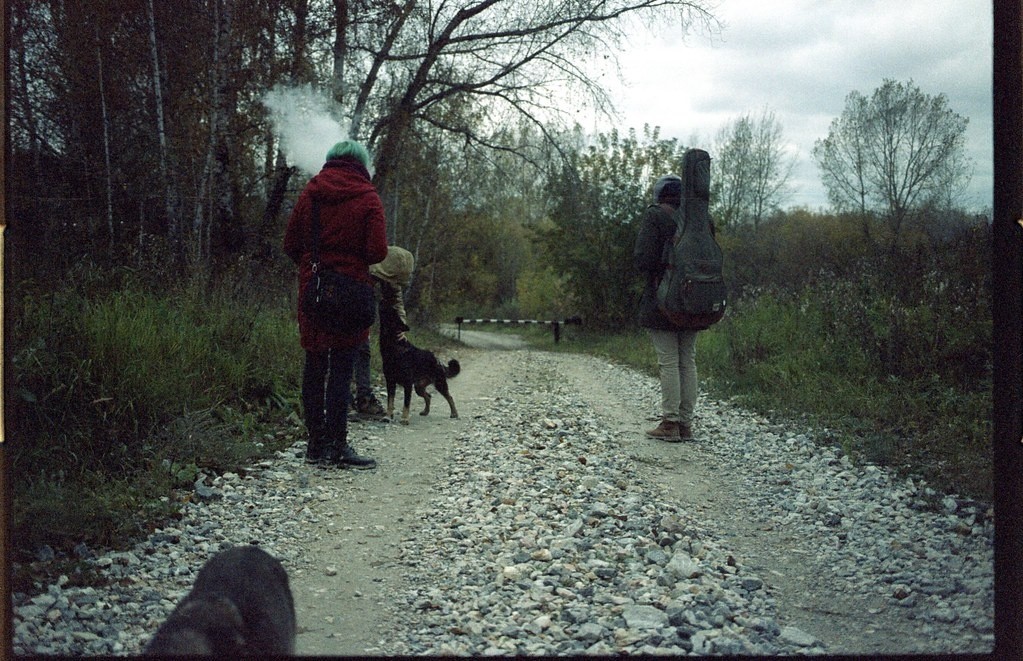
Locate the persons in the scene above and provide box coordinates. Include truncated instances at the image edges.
[344,245,416,421]
[629,178,716,443]
[283,139,392,471]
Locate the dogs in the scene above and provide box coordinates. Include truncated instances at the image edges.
[378,298,460,426]
[141,544,297,660]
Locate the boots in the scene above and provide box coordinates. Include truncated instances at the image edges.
[679,424,694,440]
[359,394,394,421]
[645,421,681,441]
[319,432,376,470]
[347,394,358,420]
[304,439,325,462]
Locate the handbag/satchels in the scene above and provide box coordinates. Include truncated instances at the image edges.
[302,272,377,336]
